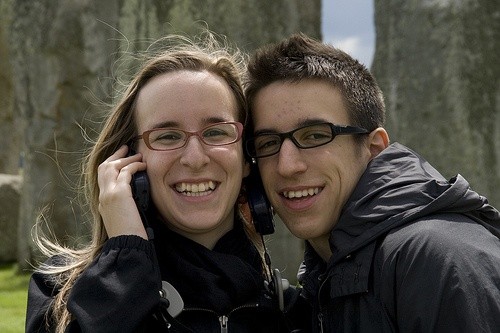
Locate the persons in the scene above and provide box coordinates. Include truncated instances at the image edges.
[23,29,322,333]
[246,29,500,332]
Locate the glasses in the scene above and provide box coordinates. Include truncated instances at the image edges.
[246,121,372,159]
[134,121,244,152]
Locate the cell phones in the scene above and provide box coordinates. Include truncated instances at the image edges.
[244,162,276,235]
[131,170,150,211]
